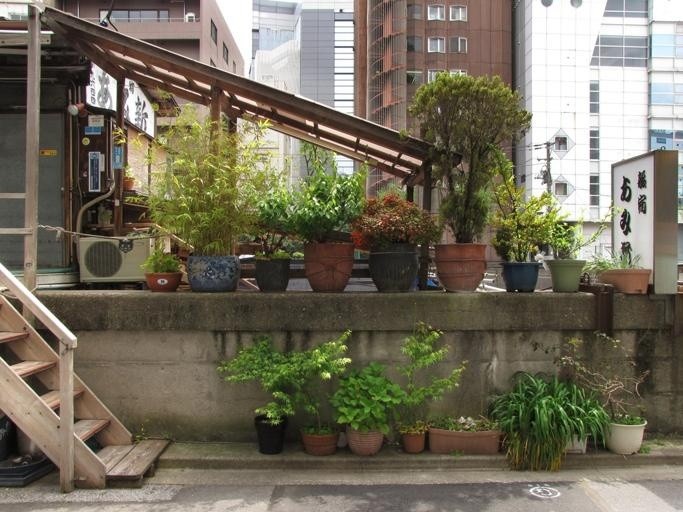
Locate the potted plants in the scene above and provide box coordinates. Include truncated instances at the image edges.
[137,247,183,292]
[391,321,470,453]
[326,362,409,458]
[254,401,289,455]
[426,371,609,473]
[111,89,290,292]
[254,184,305,292]
[527,329,649,456]
[214,327,340,455]
[256,151,367,293]
[349,194,449,292]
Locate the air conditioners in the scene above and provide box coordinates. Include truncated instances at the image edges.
[78,234,171,288]
